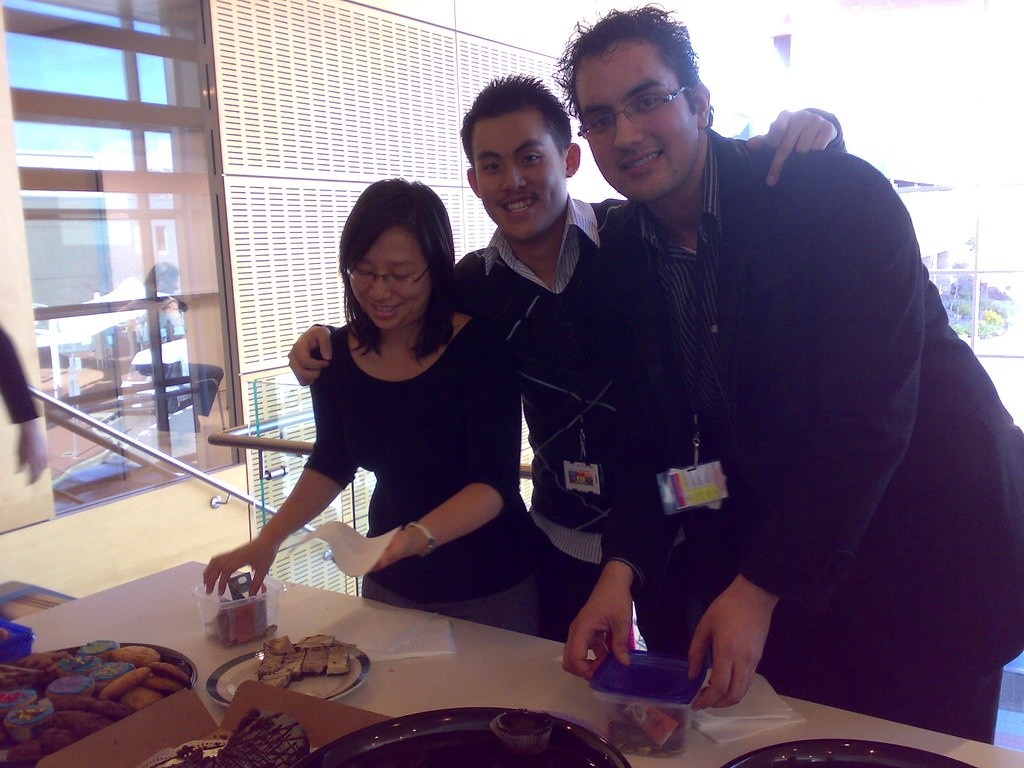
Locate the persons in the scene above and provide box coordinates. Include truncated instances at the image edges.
[556,8,1024,745]
[289,76,846,657]
[0,325,47,483]
[203,179,539,634]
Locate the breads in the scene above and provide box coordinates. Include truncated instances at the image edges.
[255,634,348,689]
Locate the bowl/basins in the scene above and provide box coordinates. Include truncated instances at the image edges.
[0,617,36,663]
[589,649,709,759]
[192,572,285,649]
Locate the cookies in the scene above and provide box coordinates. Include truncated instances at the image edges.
[0,646,194,768]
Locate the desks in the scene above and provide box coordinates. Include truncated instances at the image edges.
[0,560,1023,768]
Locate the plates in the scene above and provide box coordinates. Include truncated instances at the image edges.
[55,642,197,690]
[713,738,981,768]
[281,706,634,768]
[206,641,370,707]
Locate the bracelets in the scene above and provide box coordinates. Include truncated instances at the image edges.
[405,522,433,558]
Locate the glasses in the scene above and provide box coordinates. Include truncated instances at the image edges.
[578,85,686,139]
[345,265,429,290]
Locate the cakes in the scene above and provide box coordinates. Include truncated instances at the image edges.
[176,706,310,768]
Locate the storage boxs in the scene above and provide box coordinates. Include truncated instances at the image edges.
[590,650,707,758]
[189,576,288,649]
